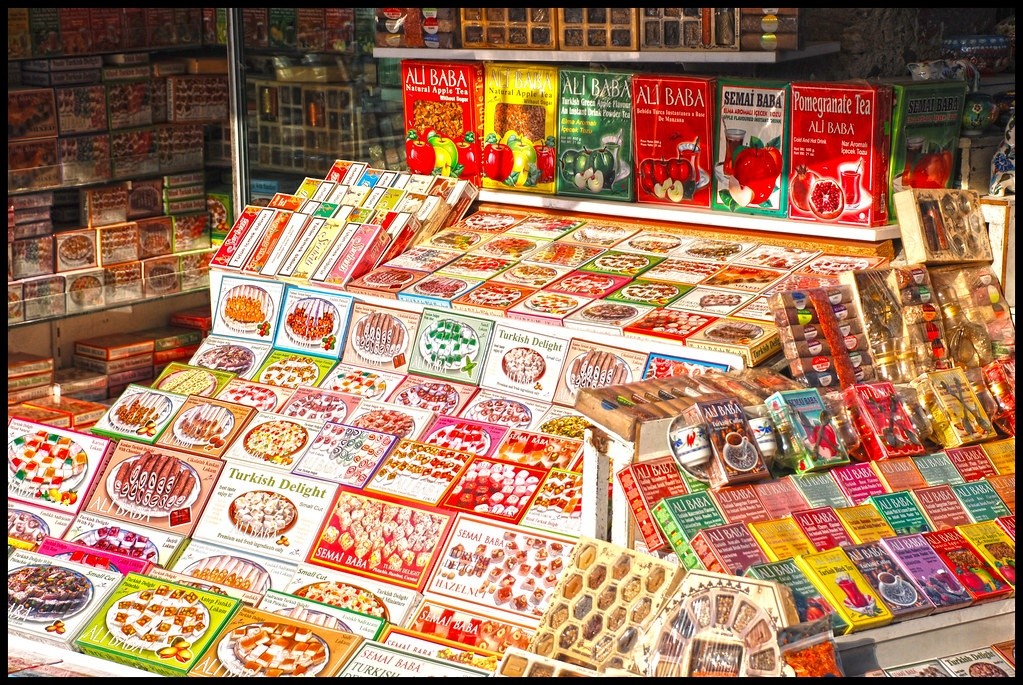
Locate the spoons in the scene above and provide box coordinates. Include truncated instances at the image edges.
[812,411,831,462]
[884,395,899,447]
[797,412,846,461]
[941,382,990,430]
[869,398,922,444]
[953,377,974,435]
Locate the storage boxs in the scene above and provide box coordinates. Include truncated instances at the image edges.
[7,5,1015,679]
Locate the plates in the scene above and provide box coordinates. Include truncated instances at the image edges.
[8,211,1012,678]
[696,167,710,190]
[614,158,631,182]
[843,186,874,216]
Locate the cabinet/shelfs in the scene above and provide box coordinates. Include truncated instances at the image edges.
[369,36,909,254]
[7,6,250,328]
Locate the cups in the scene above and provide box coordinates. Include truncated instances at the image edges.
[600,135,622,177]
[877,572,905,597]
[901,137,926,191]
[838,161,864,209]
[748,416,777,457]
[724,432,749,461]
[676,142,700,186]
[669,425,712,467]
[723,129,747,177]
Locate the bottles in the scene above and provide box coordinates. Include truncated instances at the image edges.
[936,284,991,371]
